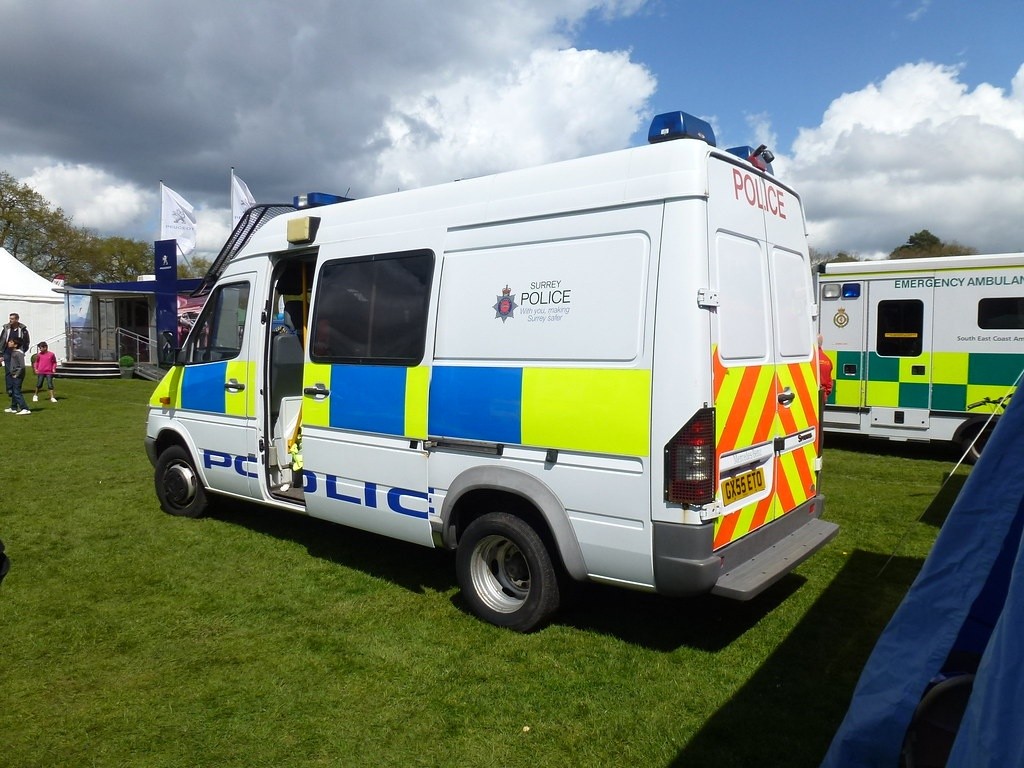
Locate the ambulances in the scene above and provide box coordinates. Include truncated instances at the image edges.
[813,253,1024,463]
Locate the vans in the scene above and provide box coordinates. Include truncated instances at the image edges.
[144,110,842,633]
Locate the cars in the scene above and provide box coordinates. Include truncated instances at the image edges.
[180,312,206,335]
[67,334,82,349]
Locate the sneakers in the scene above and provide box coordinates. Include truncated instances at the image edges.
[4,408,17,413]
[16,409,31,414]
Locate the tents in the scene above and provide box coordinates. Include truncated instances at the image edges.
[0,246,67,366]
[818,371,1024,768]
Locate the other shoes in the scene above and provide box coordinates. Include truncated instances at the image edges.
[6,390,12,397]
[51,397,57,402]
[33,395,38,401]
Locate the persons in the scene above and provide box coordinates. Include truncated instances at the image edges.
[818,334,833,405]
[269,296,294,332]
[316,303,407,358]
[180,313,190,347]
[0,313,31,415]
[33,342,58,403]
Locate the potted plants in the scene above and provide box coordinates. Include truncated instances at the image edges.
[31,353,37,374]
[119,355,135,379]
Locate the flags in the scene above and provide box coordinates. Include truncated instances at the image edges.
[162,182,197,256]
[232,175,257,231]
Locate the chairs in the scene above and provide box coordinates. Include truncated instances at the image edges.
[270,300,310,425]
